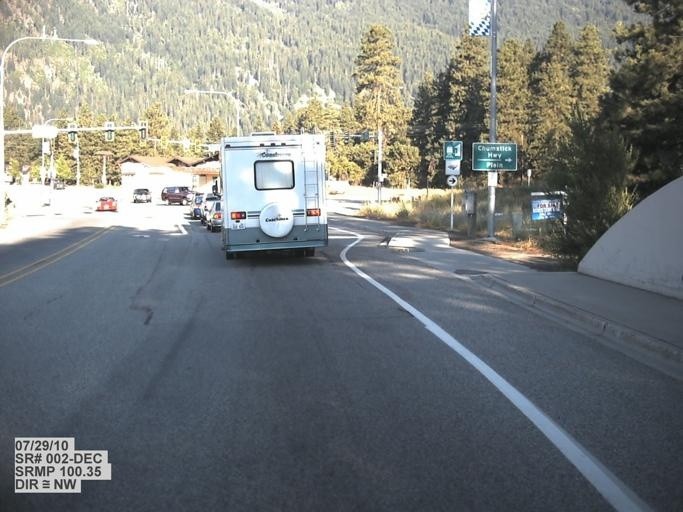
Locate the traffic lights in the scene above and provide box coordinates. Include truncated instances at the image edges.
[343,131,349,144]
[329,132,335,147]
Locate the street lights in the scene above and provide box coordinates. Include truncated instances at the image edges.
[0,36,99,92]
[41,117,73,185]
[184,89,240,137]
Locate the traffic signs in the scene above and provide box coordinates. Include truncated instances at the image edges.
[471,142,517,172]
[443,141,462,160]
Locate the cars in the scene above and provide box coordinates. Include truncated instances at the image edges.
[95,196,117,211]
[161,133,328,261]
[54,182,63,190]
[132,188,151,203]
